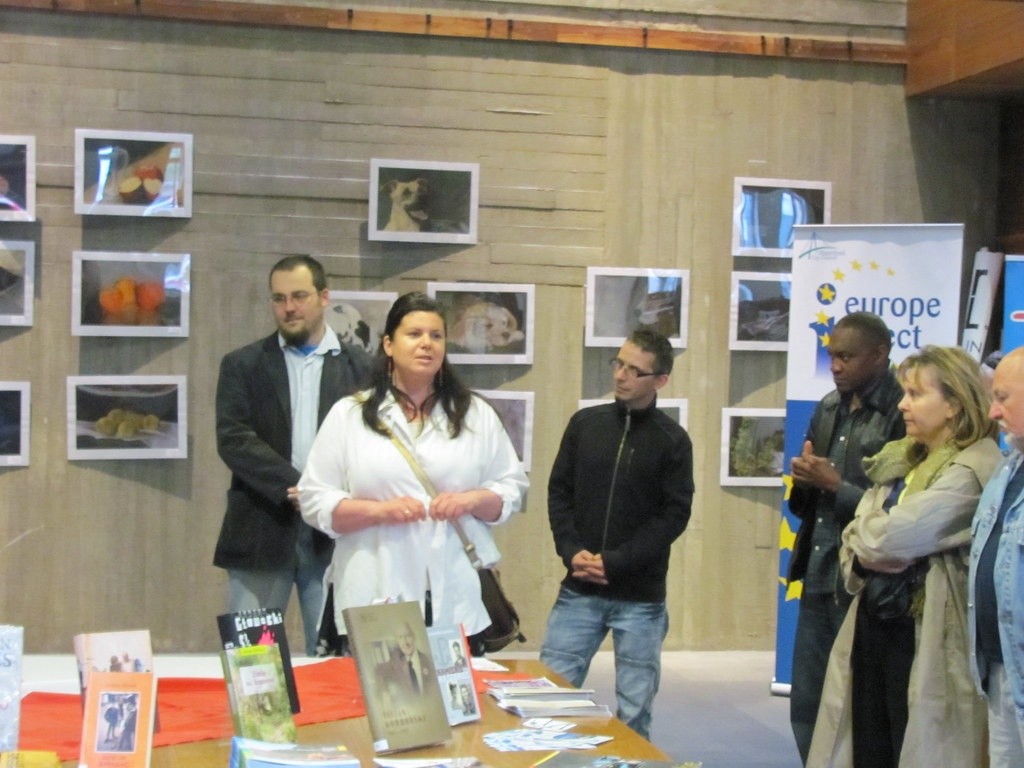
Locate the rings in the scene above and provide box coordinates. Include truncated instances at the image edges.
[404,510,410,515]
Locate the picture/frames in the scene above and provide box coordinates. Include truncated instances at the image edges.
[1,127,832,488]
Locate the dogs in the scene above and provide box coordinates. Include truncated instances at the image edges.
[381,178,432,232]
[437,291,524,353]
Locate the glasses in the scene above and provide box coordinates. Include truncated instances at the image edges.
[269,288,322,305]
[608,358,661,381]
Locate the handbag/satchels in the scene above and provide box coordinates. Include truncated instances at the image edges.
[468,566,527,656]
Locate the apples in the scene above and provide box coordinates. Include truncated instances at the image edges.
[118,166,164,202]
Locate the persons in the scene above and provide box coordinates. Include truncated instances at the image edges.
[460,685,476,716]
[449,681,462,710]
[453,642,465,665]
[395,619,434,699]
[104,693,136,751]
[805,344,1007,768]
[785,311,906,768]
[110,651,141,673]
[297,287,529,655]
[967,347,1024,768]
[212,255,378,658]
[539,330,695,741]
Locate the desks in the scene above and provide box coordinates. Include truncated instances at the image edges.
[0,653,693,768]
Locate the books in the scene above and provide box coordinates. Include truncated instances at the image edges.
[79,672,158,768]
[425,623,481,728]
[342,600,452,755]
[219,643,297,744]
[529,749,702,768]
[484,676,613,717]
[217,608,300,715]
[231,736,360,768]
[73,630,161,734]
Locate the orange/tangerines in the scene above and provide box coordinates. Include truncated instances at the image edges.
[100,279,164,310]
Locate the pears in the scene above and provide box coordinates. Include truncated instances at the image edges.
[97,409,159,437]
